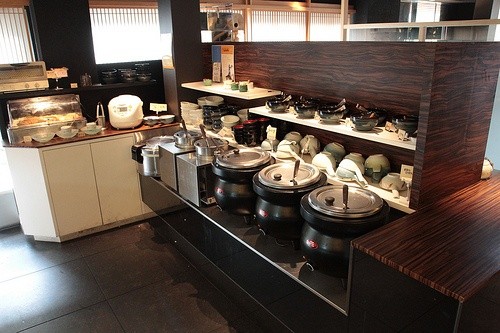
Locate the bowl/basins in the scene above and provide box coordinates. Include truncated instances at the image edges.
[143,114,175,125]
[56,129,79,138]
[391,114,418,135]
[100,63,152,83]
[81,126,103,135]
[277,119,410,199]
[179,96,279,151]
[266,93,393,131]
[30,132,55,143]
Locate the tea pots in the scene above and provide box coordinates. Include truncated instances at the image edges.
[480,157,494,179]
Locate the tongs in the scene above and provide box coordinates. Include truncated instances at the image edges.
[199,124,222,153]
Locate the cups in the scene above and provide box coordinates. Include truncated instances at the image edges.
[61,126,72,134]
[203,77,248,92]
[86,123,96,130]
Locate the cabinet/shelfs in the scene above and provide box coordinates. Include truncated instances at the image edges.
[5,125,181,243]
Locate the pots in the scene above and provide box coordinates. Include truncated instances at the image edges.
[209,148,276,215]
[173,130,229,156]
[251,166,328,238]
[299,187,390,266]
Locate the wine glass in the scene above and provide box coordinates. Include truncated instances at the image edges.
[51,67,68,90]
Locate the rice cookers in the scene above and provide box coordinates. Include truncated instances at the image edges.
[131,135,177,177]
[107,94,144,131]
